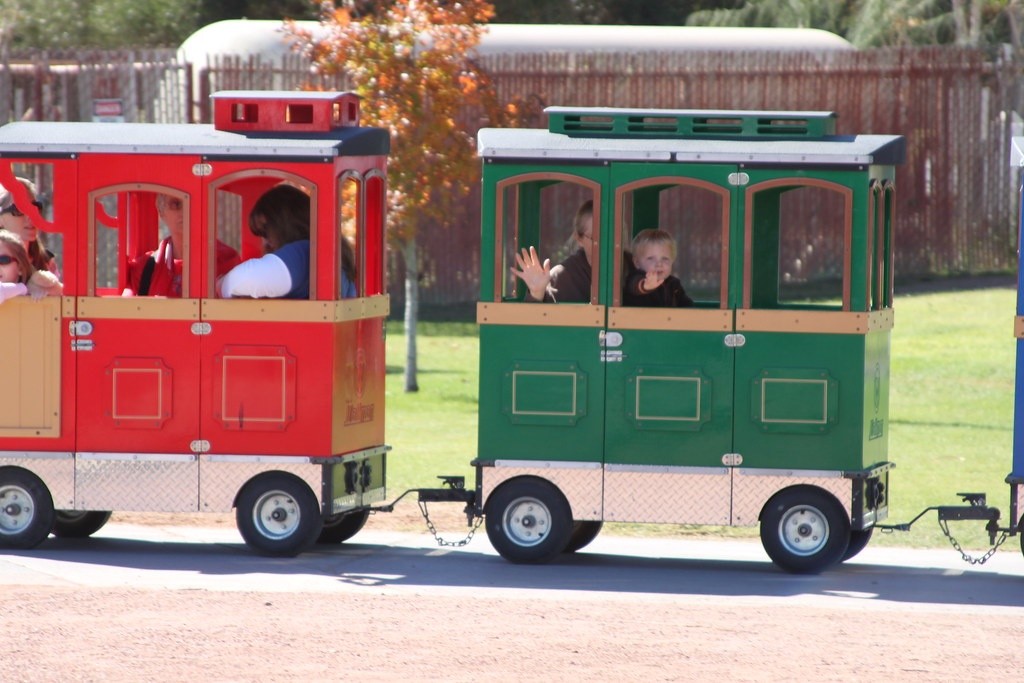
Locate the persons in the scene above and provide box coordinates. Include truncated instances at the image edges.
[509,198,635,305]
[213,184,359,300]
[119,190,246,302]
[0,228,65,301]
[623,228,697,309]
[0,175,60,280]
[18,85,65,206]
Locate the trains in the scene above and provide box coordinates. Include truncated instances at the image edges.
[1,88,1023,576]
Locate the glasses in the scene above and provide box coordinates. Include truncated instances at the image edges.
[165,198,183,211]
[584,233,595,242]
[0,253,19,267]
[0,201,44,219]
